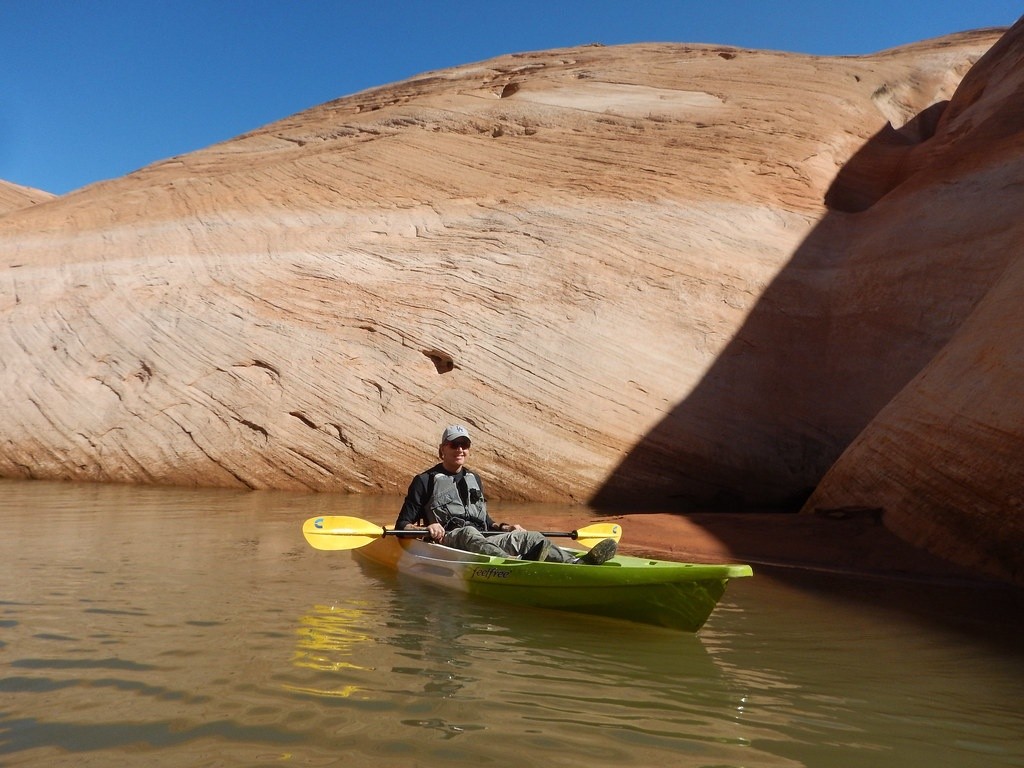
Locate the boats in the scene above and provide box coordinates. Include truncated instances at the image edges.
[353,520,755,634]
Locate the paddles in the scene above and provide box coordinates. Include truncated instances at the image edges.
[301,514,623,551]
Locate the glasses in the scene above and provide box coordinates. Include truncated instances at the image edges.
[443,441,470,450]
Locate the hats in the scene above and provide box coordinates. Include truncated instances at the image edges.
[441,426,472,444]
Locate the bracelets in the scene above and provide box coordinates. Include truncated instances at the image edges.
[499,522,509,531]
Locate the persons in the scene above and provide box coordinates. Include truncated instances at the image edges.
[394,426,617,565]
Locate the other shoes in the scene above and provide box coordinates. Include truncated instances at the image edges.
[521,539,551,563]
[574,539,617,565]
